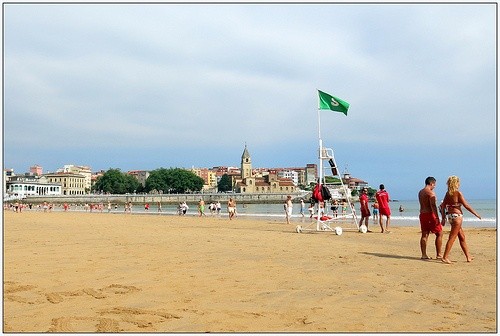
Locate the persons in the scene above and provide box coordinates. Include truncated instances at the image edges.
[84,201,134,214]
[178,201,189,216]
[198,198,207,217]
[399,204,404,212]
[284,195,292,225]
[441,176,481,263]
[300,200,305,217]
[372,201,379,221]
[5,200,71,212]
[308,205,314,218]
[208,201,221,215]
[372,184,392,233]
[332,199,338,218]
[419,177,443,260]
[320,200,324,217]
[341,199,347,216]
[145,202,149,212]
[359,188,371,232]
[228,198,238,220]
[158,201,163,212]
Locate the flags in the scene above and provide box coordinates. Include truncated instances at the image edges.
[318,90,350,116]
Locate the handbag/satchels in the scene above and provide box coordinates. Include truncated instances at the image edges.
[322,186,331,200]
[313,183,322,200]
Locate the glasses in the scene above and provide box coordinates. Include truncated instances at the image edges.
[364,190,367,192]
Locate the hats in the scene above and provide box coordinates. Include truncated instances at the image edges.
[379,184,385,189]
